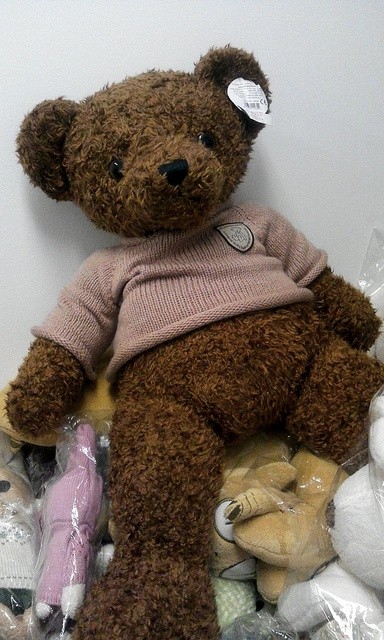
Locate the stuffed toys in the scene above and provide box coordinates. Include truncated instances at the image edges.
[1,423,103,640]
[221,612,299,640]
[1,359,350,604]
[4,46,383,639]
[275,395,384,640]
